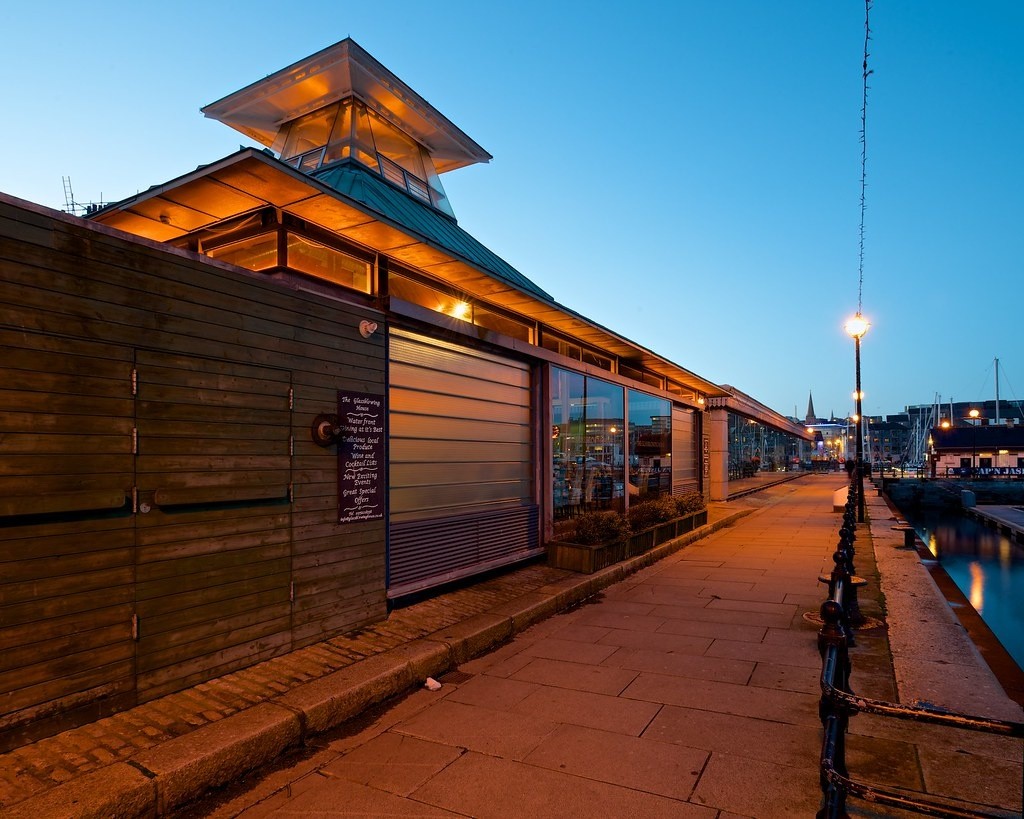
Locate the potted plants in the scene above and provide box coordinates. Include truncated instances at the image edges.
[546,489,709,574]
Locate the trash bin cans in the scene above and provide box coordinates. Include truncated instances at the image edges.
[864,462,872,476]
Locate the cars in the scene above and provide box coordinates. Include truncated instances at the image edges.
[554,457,608,467]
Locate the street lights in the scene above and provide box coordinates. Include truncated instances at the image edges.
[969,410,979,478]
[610,428,616,469]
[845,313,870,524]
